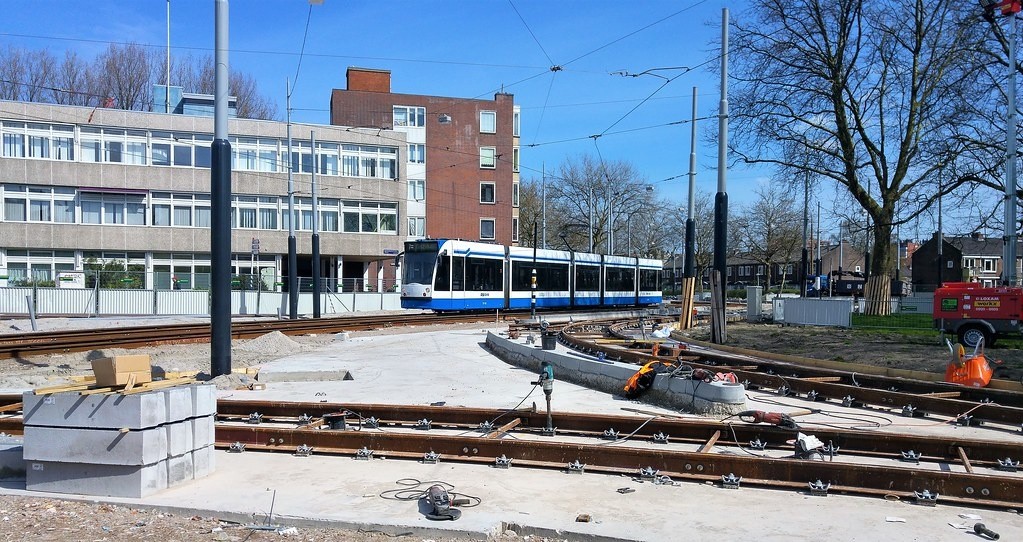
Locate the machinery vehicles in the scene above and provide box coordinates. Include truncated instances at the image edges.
[933,275,1023,348]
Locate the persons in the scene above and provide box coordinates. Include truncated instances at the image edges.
[172,277,181,290]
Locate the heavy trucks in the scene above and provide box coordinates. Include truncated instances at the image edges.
[802,270,912,298]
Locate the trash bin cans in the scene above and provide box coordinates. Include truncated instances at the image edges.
[907,282,912,295]
[891,280,908,297]
[541,331,556,350]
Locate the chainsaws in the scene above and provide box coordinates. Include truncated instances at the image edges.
[427,486,470,521]
[738,410,801,431]
[686,369,713,380]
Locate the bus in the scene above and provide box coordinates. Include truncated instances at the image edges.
[381,235,664,317]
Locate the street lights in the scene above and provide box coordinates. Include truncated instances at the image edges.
[606,185,655,255]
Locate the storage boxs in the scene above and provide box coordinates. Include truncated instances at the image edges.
[91,355,151,386]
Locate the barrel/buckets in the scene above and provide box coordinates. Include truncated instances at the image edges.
[542,335,556,350]
